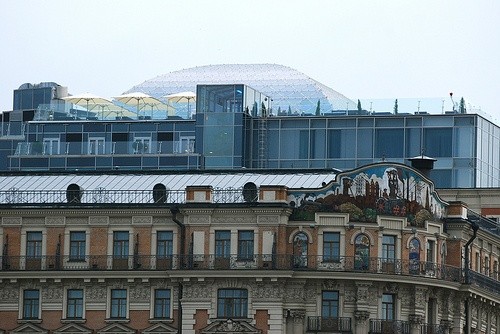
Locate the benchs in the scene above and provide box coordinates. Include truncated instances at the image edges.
[53,112,74,120]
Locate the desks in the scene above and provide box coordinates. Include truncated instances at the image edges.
[87,115,195,120]
[277,110,458,117]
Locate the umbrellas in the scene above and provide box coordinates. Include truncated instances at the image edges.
[60,91,197,120]
